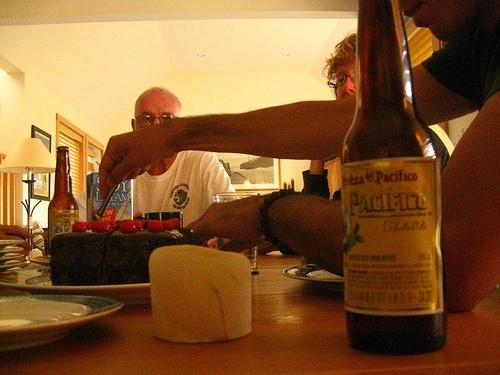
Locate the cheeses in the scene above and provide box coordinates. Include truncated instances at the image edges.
[149,244,253,342]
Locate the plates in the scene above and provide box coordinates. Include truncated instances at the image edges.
[281,263,345,292]
[0,293,127,352]
[0,265,153,305]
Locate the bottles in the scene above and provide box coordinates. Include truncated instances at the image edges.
[342,0,446,356]
[47,145,79,258]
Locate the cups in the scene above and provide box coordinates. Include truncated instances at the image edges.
[210,193,262,271]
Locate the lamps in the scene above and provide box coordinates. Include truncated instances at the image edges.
[0,138,56,252]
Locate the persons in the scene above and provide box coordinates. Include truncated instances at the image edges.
[77,85,242,229]
[99,0,500,314]
[302,32,456,201]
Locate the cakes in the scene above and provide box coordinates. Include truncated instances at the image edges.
[49,218,207,287]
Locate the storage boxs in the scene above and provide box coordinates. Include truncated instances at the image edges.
[86,173,133,222]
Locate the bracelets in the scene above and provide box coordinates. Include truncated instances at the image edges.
[258,189,304,256]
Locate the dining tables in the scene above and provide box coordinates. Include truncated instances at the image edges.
[0,250,500,375]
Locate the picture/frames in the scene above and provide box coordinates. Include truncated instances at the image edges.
[30,125,51,201]
[212,152,281,191]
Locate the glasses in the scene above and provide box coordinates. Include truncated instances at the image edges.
[326,71,357,89]
[135,112,178,128]
